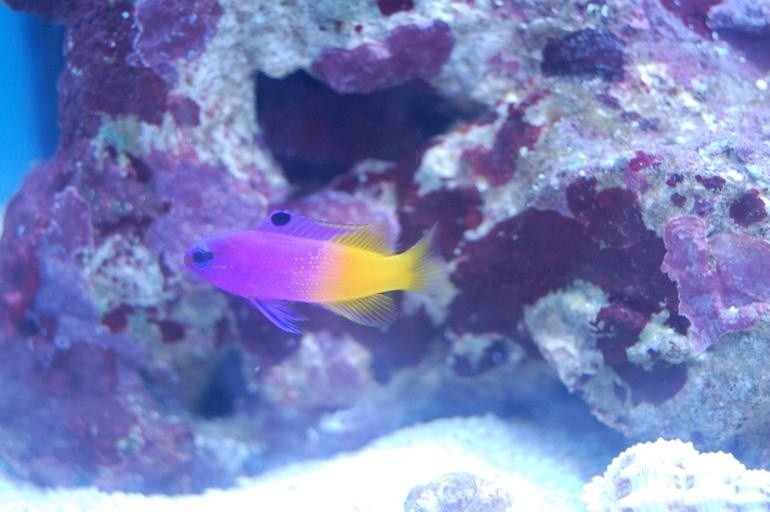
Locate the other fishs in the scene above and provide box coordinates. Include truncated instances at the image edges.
[183,210,444,335]
